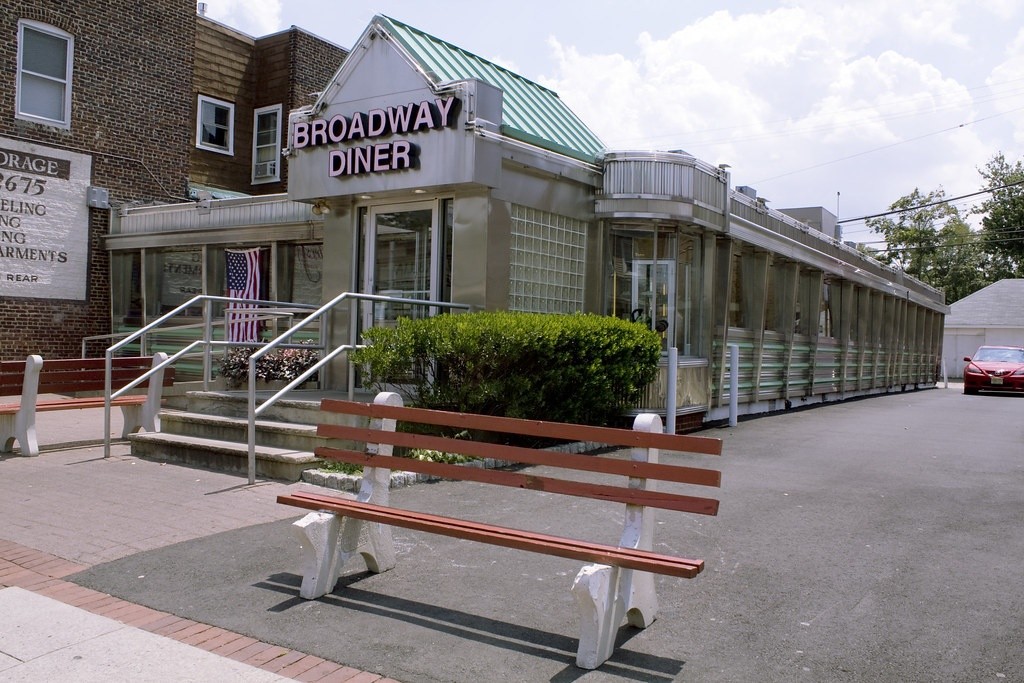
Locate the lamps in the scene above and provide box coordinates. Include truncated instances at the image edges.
[320,200,331,215]
[312,202,322,215]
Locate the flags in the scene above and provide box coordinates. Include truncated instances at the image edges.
[225,246,263,343]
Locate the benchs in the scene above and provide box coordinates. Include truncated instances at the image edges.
[0,352,177,457]
[275,390,724,670]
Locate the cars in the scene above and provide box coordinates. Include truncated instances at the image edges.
[963,345,1024,395]
